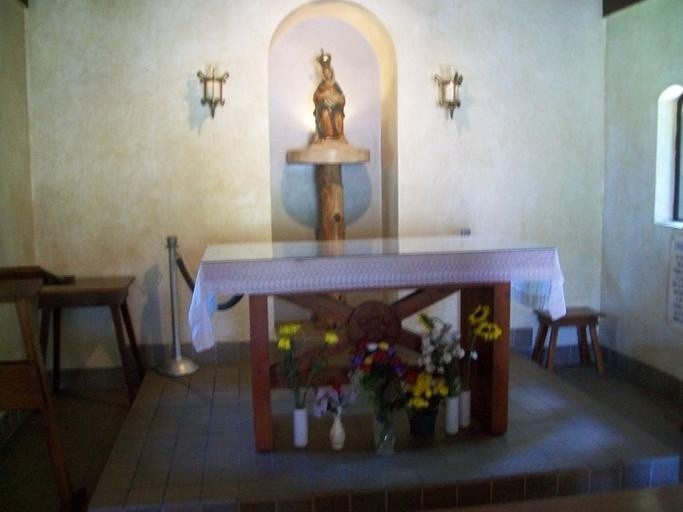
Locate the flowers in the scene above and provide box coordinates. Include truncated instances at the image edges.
[278,303,502,423]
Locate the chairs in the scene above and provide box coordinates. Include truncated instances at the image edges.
[0,267,74,512]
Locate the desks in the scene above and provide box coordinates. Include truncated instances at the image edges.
[202,234,557,453]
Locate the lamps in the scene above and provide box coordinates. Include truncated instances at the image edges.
[198,65,229,117]
[435,70,463,120]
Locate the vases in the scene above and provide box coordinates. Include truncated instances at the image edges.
[292,392,472,453]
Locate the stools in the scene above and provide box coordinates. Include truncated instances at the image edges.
[39,277,144,404]
[531,306,608,374]
